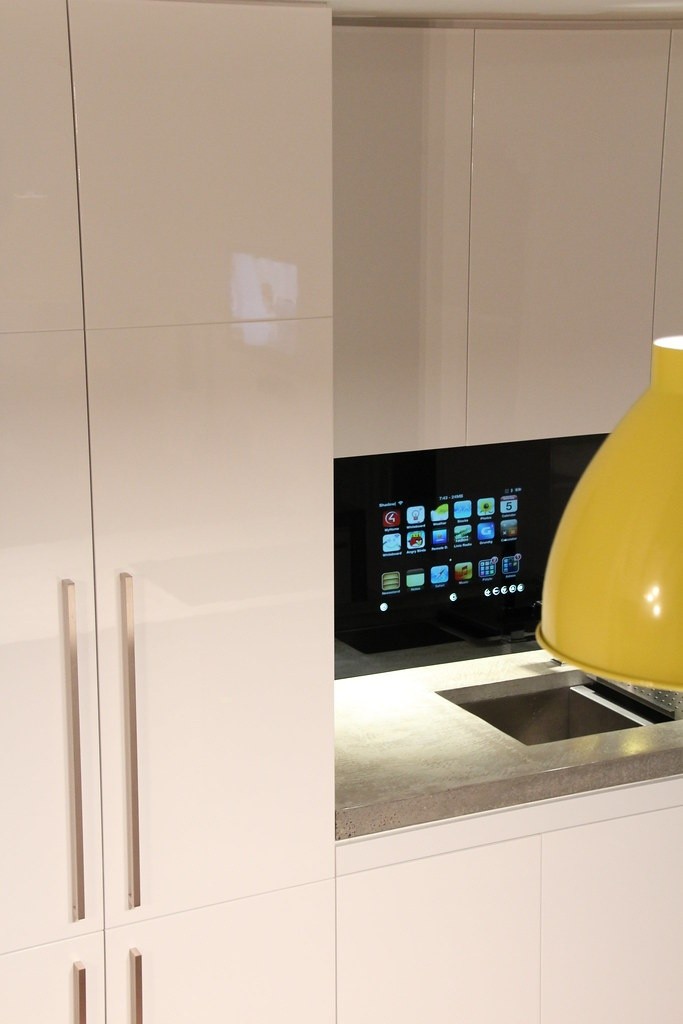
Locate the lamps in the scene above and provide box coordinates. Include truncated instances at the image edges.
[534,336,683,694]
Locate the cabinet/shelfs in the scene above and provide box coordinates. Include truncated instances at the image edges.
[334,17,683,459]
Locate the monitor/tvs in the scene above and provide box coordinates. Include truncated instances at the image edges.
[333,433,610,655]
